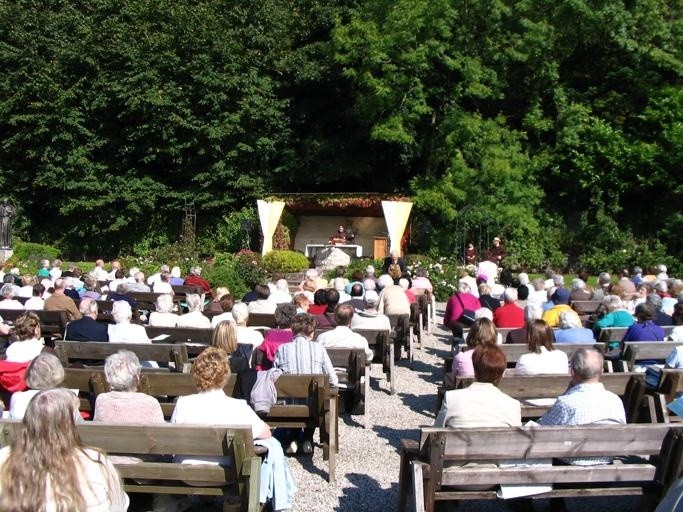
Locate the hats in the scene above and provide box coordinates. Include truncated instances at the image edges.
[476,273,488,282]
[551,288,570,305]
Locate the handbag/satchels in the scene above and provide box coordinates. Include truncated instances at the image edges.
[455,291,476,324]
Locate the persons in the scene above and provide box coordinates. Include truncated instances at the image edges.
[2,274,18,296]
[0,284,21,310]
[176,290,209,326]
[10,266,21,275]
[128,267,139,280]
[149,263,169,285]
[274,314,341,455]
[434,344,521,491]
[0,313,12,340]
[505,278,523,302]
[599,266,683,295]
[211,294,238,327]
[466,241,479,265]
[0,386,130,511]
[10,351,80,420]
[671,301,683,325]
[466,306,494,326]
[169,267,182,286]
[148,295,180,326]
[314,303,373,360]
[292,293,310,312]
[578,271,590,282]
[20,274,35,295]
[51,257,64,269]
[547,273,564,297]
[451,317,498,378]
[310,289,329,314]
[213,321,238,352]
[153,272,173,292]
[444,283,478,328]
[493,287,525,328]
[492,270,513,289]
[82,275,102,299]
[90,259,108,279]
[297,254,434,289]
[112,283,130,297]
[536,348,627,505]
[543,288,581,328]
[38,259,49,278]
[68,297,108,342]
[329,224,350,242]
[258,301,298,355]
[37,259,51,270]
[247,283,277,314]
[377,274,412,363]
[24,284,46,310]
[231,303,264,346]
[515,284,542,323]
[646,296,673,326]
[207,286,231,319]
[70,267,84,288]
[533,276,549,291]
[518,320,569,374]
[554,310,594,343]
[51,260,63,279]
[6,312,45,360]
[653,345,683,369]
[326,286,341,308]
[0,262,7,285]
[459,268,480,300]
[109,269,125,288]
[275,276,291,297]
[93,348,167,486]
[43,279,83,325]
[622,304,666,343]
[572,277,591,299]
[518,270,535,291]
[108,260,122,280]
[350,289,391,354]
[487,237,506,265]
[130,271,150,293]
[109,299,149,343]
[186,264,210,290]
[172,345,271,503]
[596,293,634,328]
[478,281,501,308]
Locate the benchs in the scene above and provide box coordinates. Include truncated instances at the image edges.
[172,284,194,304]
[199,311,276,328]
[0,365,338,487]
[461,326,561,342]
[569,299,602,321]
[654,368,683,422]
[391,288,434,365]
[326,345,367,420]
[598,324,678,343]
[128,292,160,309]
[77,299,174,318]
[399,423,670,511]
[317,327,396,398]
[619,342,683,372]
[146,327,215,344]
[437,373,658,424]
[2,418,263,512]
[446,342,613,374]
[0,307,68,337]
[54,341,255,371]
